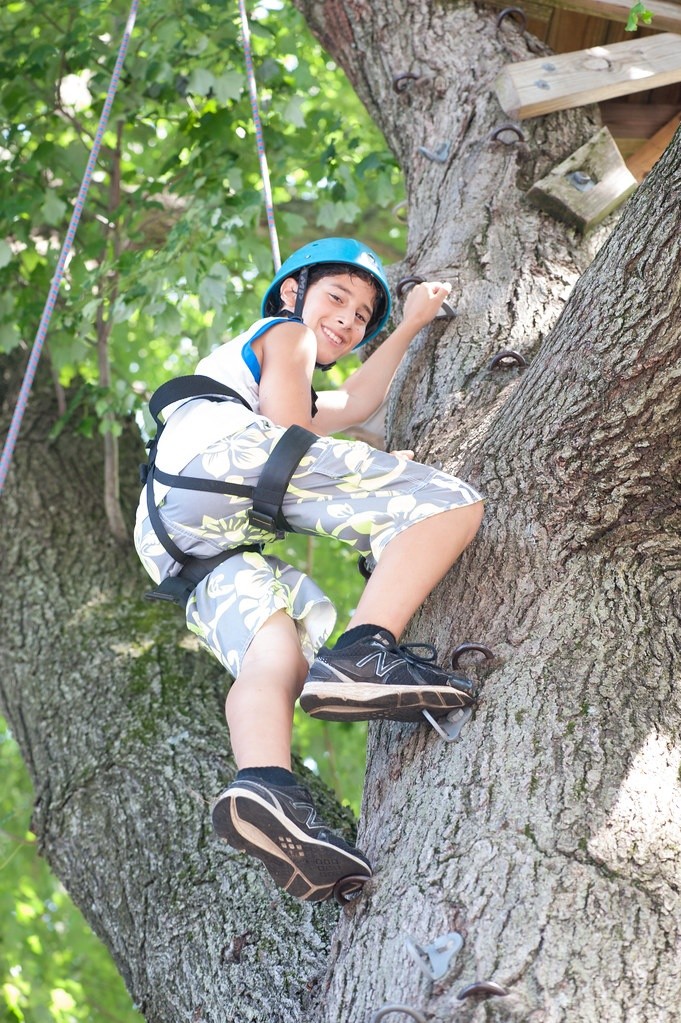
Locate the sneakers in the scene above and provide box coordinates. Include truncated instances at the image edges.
[298,631,477,722]
[209,775,374,904]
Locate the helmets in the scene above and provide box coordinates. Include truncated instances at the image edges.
[259,236,393,370]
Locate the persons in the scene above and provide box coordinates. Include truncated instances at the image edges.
[134,238,487,907]
